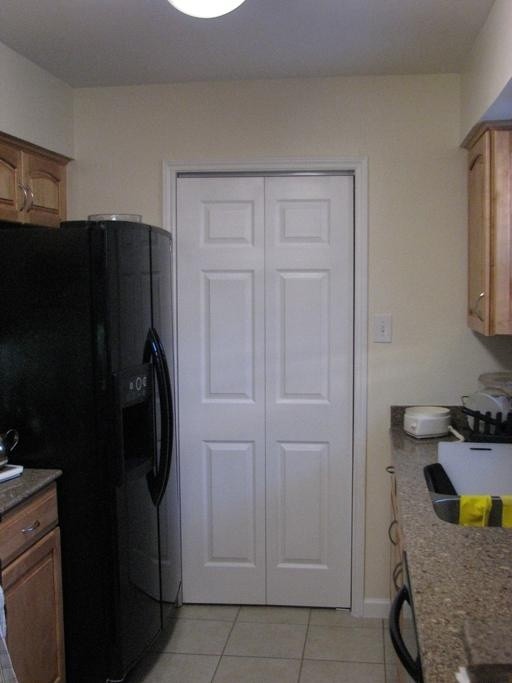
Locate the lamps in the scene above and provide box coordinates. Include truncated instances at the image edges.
[168,0,246,18]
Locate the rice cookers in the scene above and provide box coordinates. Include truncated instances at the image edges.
[403,405,452,439]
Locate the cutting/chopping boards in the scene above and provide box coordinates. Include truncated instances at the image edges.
[437,441,511,498]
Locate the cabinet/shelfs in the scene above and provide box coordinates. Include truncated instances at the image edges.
[0,131,74,228]
[390,447,421,683]
[0,481,66,683]
[460,119,511,337]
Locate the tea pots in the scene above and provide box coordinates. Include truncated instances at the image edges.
[0,425,20,469]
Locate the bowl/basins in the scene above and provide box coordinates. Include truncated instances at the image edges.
[476,372,512,397]
[461,388,512,433]
[88,212,144,224]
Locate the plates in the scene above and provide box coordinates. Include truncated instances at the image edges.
[0,464,27,482]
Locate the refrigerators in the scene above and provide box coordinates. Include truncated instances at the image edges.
[0,220,184,683]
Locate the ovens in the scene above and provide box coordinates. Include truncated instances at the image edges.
[388,549,423,682]
